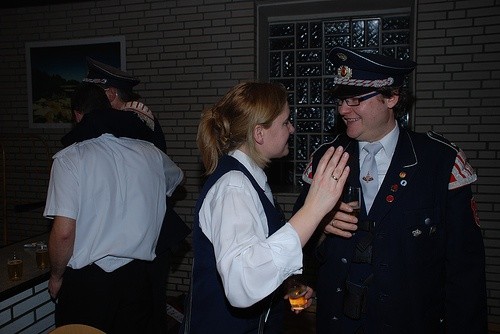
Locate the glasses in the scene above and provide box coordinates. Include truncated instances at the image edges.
[333,89,388,107]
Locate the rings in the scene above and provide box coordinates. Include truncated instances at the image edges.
[331,175,338,181]
[332,220,336,227]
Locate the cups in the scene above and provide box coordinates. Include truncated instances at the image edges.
[6,252,23,281]
[287,279,308,311]
[338,187,361,234]
[35,244,48,268]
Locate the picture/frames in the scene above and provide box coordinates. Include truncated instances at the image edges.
[25,36,126,129]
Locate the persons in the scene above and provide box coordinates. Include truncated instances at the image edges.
[43,83,184,333]
[188,81,350,333]
[293,47,488,333]
[61,58,192,264]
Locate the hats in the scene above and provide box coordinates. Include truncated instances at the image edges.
[81,58,141,96]
[327,46,416,99]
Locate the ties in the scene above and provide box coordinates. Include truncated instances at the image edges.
[359,143,383,215]
